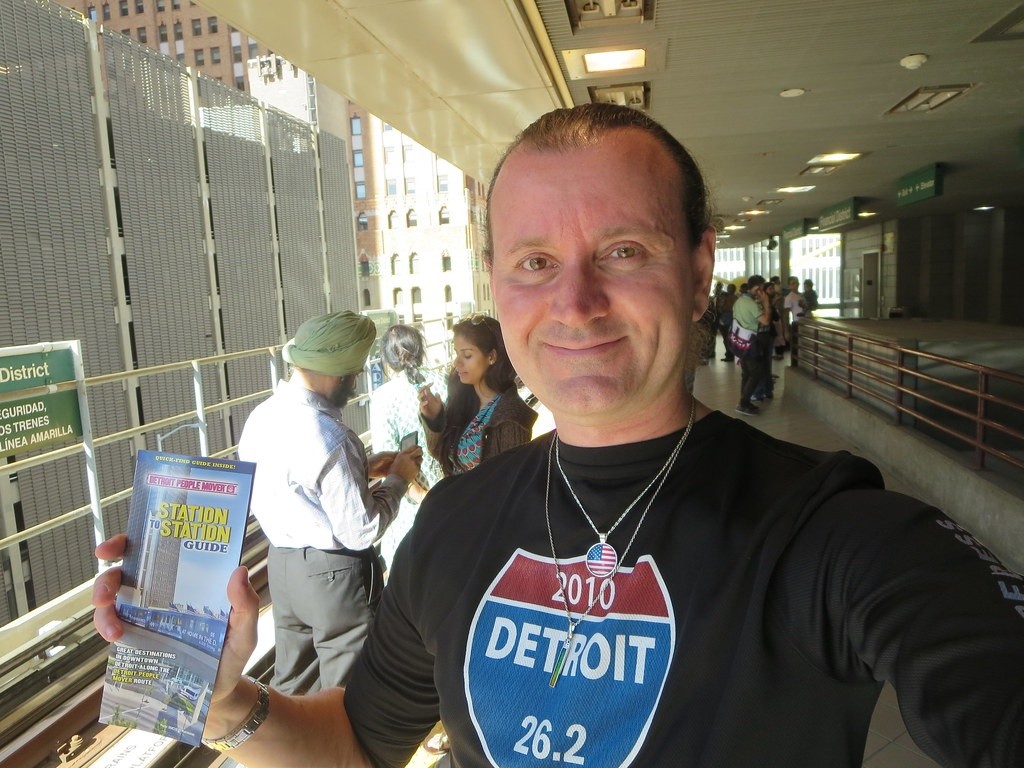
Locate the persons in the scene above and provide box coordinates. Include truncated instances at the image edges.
[420,315,539,479]
[237,310,422,698]
[703,276,818,415]
[783,277,806,365]
[717,284,738,362]
[370,324,448,585]
[90,103,1024,768]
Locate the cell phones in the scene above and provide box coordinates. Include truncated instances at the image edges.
[755,287,762,295]
[401,431,418,452]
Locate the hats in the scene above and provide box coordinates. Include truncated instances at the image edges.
[282,309,376,376]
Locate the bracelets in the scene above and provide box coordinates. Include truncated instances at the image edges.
[201,674,269,751]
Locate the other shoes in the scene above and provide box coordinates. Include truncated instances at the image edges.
[750,394,770,400]
[735,404,760,416]
[721,356,735,362]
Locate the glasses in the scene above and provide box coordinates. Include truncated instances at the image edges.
[458,312,496,338]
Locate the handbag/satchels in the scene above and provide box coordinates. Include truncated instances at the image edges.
[725,319,761,361]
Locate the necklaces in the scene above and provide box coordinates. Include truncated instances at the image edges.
[546,402,694,688]
[555,400,694,577]
[472,396,498,428]
